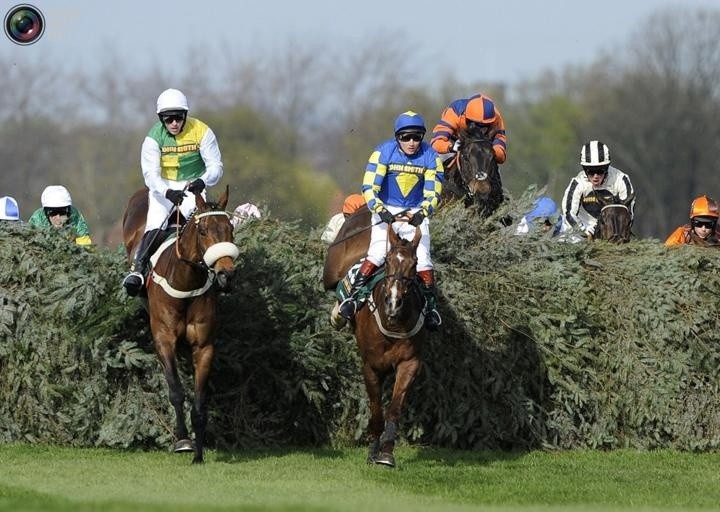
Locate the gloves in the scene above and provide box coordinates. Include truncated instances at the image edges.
[186,178,206,195]
[377,208,397,226]
[449,138,462,153]
[407,210,427,227]
[164,188,189,208]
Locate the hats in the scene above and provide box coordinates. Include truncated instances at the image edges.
[464,94,499,124]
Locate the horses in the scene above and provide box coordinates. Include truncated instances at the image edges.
[121,184,238,465]
[439,123,514,233]
[323,203,433,468]
[589,186,638,245]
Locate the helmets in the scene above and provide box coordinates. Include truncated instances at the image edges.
[689,194,719,219]
[40,184,73,209]
[156,88,189,114]
[393,109,427,133]
[580,141,611,166]
[232,202,261,227]
[0,194,20,221]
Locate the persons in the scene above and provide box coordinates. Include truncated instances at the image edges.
[122,88,223,295]
[513,197,573,237]
[0,195,19,220]
[29,185,91,245]
[663,196,720,246]
[329,110,445,333]
[562,139,636,243]
[321,194,366,244]
[430,93,507,170]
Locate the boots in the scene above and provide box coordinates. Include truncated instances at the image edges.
[422,284,440,326]
[125,230,151,298]
[337,273,368,323]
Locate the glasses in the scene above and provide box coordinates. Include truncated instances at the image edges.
[585,167,605,175]
[46,208,69,216]
[692,219,715,229]
[397,132,423,141]
[164,114,184,124]
